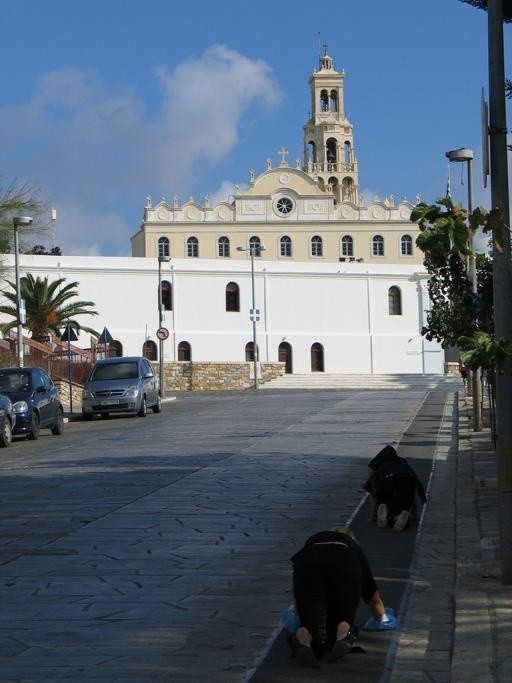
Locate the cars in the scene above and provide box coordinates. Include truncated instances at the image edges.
[0,367,64,447]
[82,357,161,421]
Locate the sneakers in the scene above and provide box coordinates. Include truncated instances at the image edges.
[376,503,388,529]
[392,510,410,532]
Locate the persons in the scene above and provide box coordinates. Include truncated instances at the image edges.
[368,445,418,532]
[290,528,385,667]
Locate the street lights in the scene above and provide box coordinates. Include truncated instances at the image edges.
[13,216,34,367]
[446,148,484,432]
[237,245,265,389]
[158,256,184,397]
